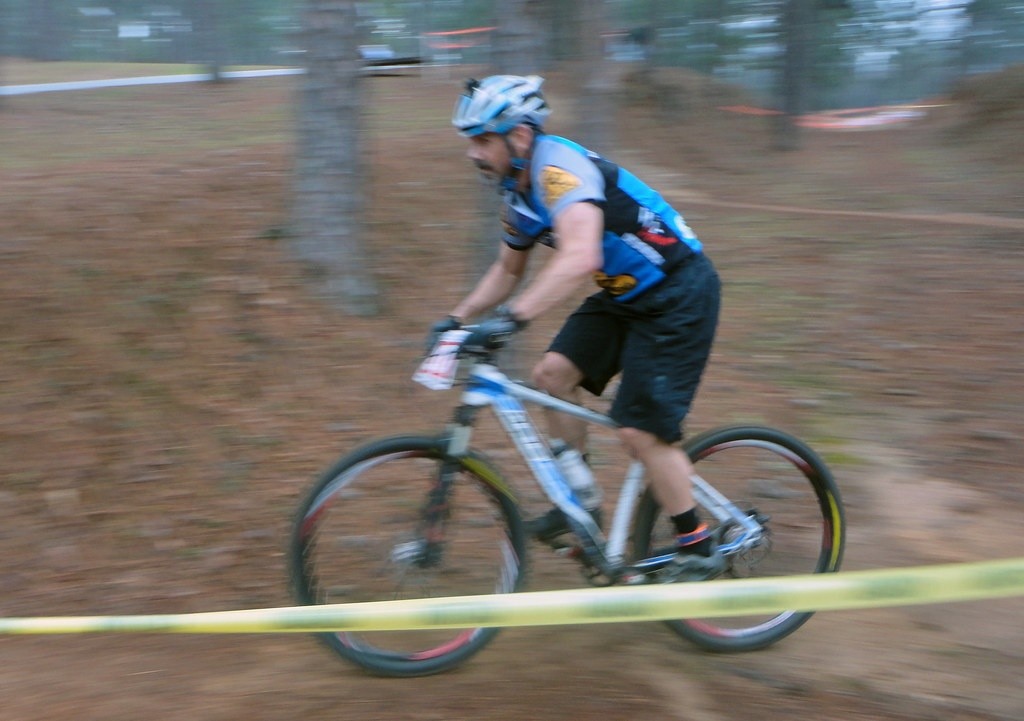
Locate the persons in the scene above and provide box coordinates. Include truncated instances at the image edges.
[423,72,731,588]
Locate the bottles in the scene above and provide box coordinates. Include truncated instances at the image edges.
[550,439,604,514]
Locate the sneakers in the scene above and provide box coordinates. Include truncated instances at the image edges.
[652,538,727,584]
[522,506,603,546]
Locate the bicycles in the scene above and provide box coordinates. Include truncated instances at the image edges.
[290,329,845,682]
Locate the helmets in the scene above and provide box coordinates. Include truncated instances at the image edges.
[451,74,552,138]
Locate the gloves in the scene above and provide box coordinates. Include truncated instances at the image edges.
[457,304,528,352]
[424,314,461,350]
